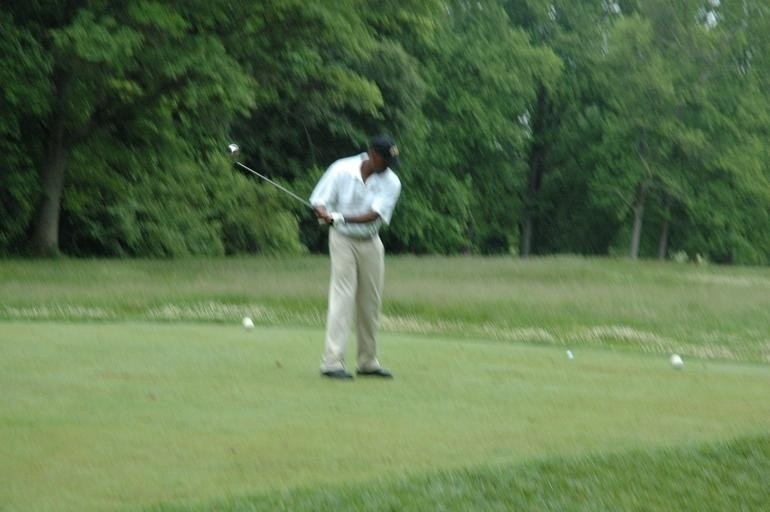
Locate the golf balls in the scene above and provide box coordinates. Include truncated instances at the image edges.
[242,317,255,331]
[670,354,683,369]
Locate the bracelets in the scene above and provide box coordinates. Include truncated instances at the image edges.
[330,211,346,226]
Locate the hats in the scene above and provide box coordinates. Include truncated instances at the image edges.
[370,136,402,169]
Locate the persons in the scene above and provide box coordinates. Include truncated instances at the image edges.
[308,133,402,380]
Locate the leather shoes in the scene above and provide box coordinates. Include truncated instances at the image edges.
[356,369,392,377]
[322,370,353,379]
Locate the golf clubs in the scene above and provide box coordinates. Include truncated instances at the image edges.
[227,144,317,213]
